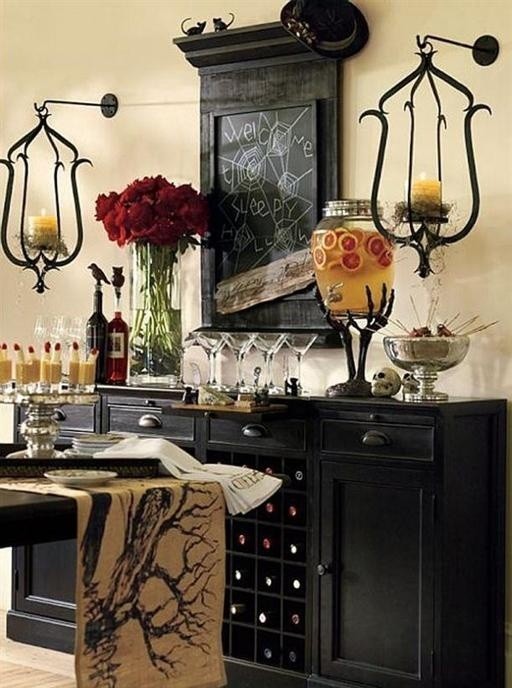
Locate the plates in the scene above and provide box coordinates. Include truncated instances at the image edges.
[64,431,138,456]
[42,468,119,486]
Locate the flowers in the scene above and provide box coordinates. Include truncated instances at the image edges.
[95,173,210,375]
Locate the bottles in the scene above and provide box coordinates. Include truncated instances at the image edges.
[87,283,127,388]
[312,200,395,319]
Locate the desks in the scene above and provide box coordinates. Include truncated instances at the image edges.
[0,473,226,549]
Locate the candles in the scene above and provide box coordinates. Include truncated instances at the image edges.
[27,215,57,243]
[404,178,442,204]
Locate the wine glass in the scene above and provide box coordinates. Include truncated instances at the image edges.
[192,332,318,395]
[381,336,469,403]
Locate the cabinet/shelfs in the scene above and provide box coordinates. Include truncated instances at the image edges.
[7,388,506,688]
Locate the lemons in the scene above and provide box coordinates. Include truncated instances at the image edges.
[312,226,394,273]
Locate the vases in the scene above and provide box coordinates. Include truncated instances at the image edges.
[125,235,184,387]
